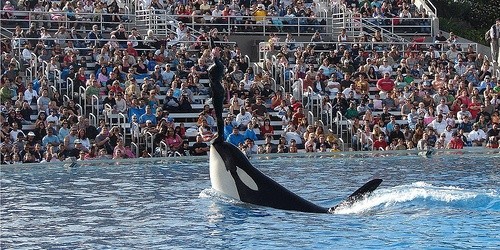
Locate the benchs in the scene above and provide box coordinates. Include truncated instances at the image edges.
[10,53,436,153]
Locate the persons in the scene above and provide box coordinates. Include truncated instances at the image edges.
[0,0,500,165]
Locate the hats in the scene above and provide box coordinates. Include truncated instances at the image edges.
[74,139,82,144]
[138,57,143,60]
[28,132,35,136]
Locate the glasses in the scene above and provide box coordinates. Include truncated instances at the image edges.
[117,95,121,96]
[239,85,244,86]
[155,68,159,69]
[71,129,77,131]
[166,66,170,67]
[109,93,114,95]
[383,61,387,62]
[385,75,389,77]
[120,27,123,28]
[257,99,261,100]
[310,137,314,138]
